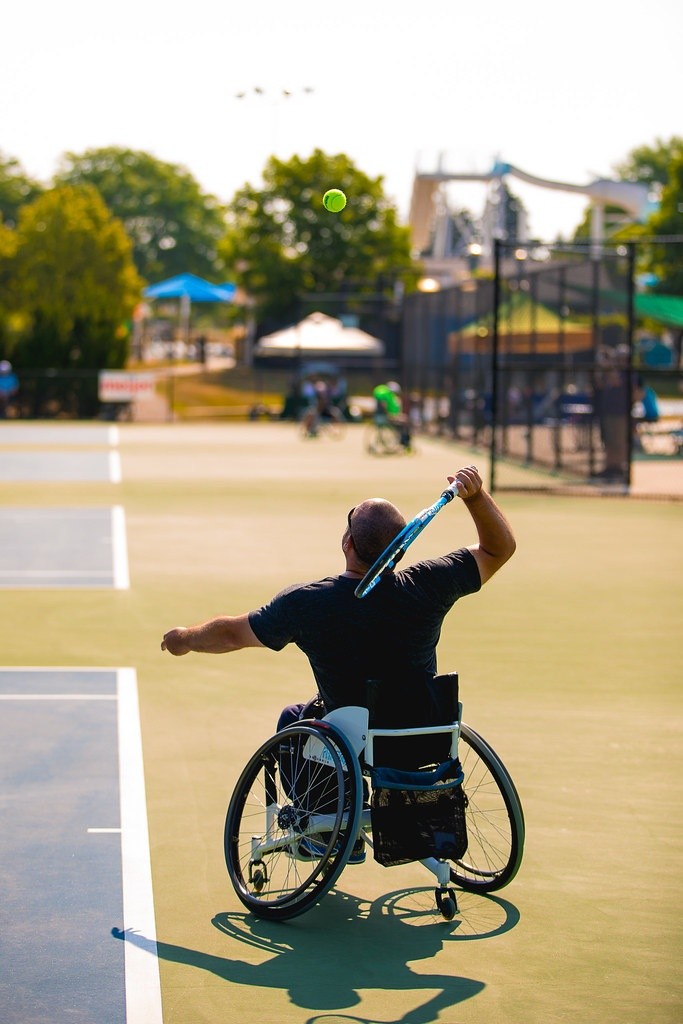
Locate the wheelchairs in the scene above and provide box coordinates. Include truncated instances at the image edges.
[223,672,527,923]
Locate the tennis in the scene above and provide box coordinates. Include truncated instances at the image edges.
[323,188,347,213]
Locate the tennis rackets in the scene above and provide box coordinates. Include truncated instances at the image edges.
[354,469,475,599]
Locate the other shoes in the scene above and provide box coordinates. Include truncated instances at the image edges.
[283,834,365,865]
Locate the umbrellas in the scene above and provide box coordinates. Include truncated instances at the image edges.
[253,310,388,423]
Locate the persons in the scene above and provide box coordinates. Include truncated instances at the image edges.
[366,381,399,456]
[0,357,21,418]
[160,464,516,867]
[629,377,661,449]
